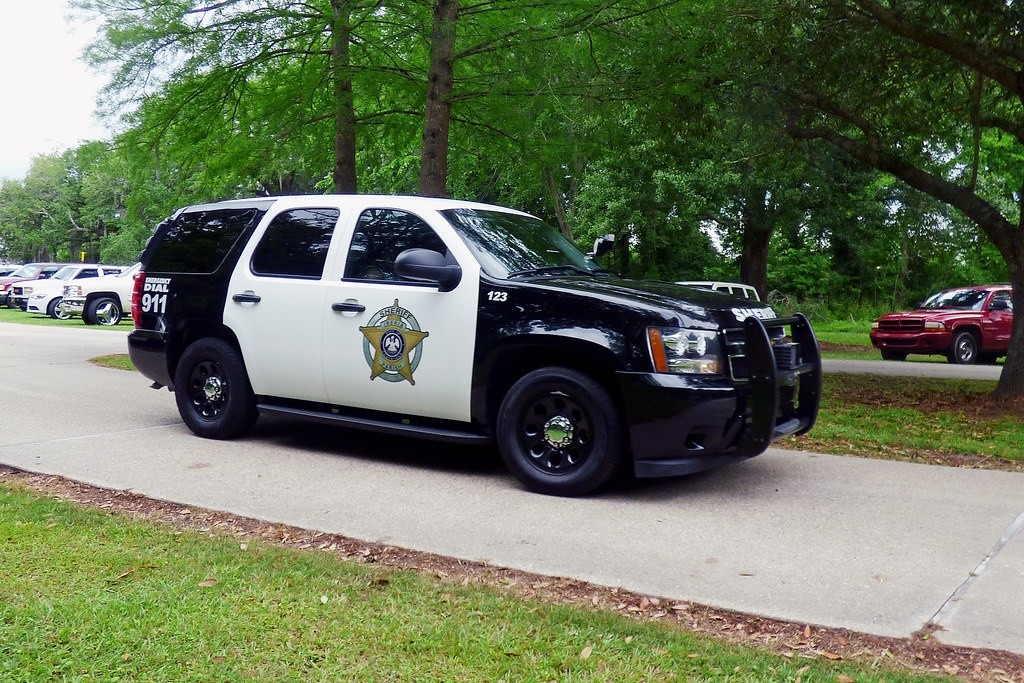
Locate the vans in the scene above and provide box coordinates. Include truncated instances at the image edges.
[673,280,761,301]
[26,264,130,320]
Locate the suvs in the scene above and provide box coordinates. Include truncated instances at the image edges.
[0,262,65,309]
[125,191,823,499]
[7,266,69,312]
[869,284,1015,364]
[61,261,142,327]
